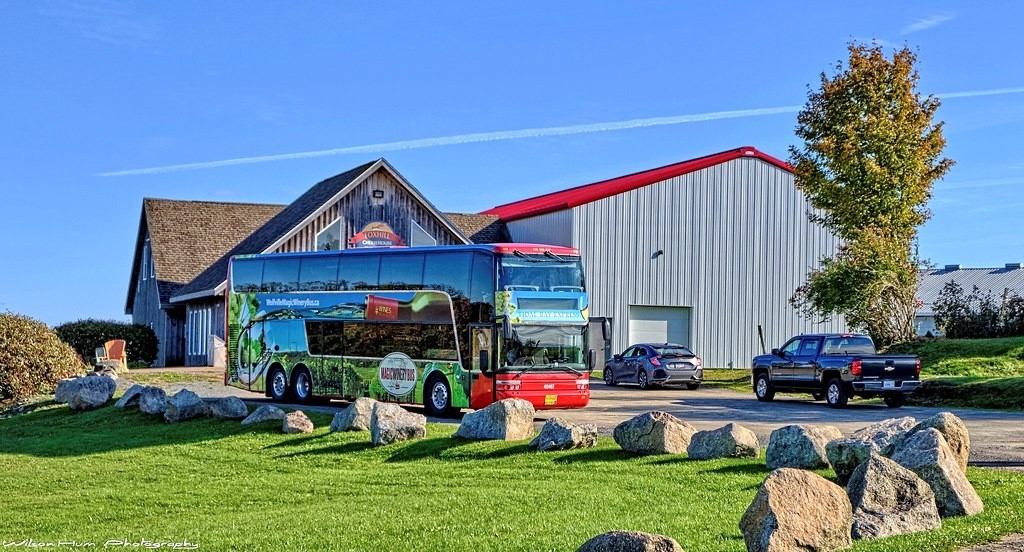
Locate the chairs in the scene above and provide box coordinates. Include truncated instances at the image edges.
[96,347,106,363]
[104,339,126,361]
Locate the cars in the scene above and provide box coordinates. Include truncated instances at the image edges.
[604,342,704,391]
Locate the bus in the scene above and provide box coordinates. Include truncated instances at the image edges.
[223,243,611,417]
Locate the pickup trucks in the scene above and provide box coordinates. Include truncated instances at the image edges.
[750,332,922,409]
